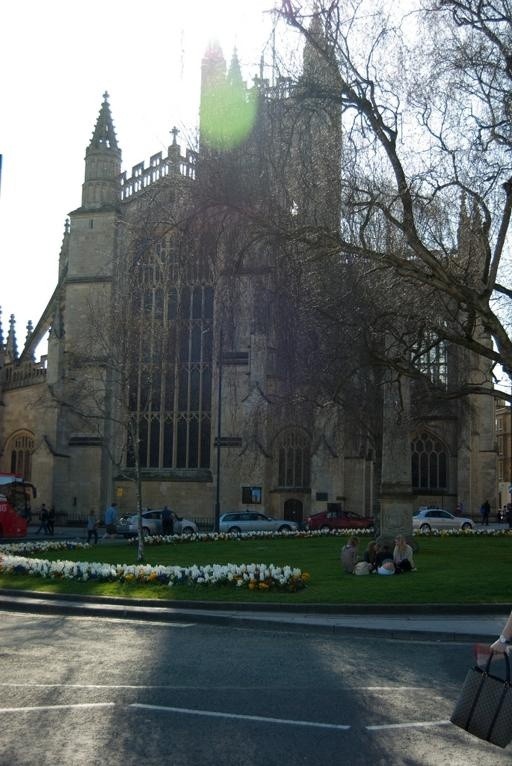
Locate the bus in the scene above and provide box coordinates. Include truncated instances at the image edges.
[1,473,39,541]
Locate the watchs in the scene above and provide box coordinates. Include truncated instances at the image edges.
[499,635,510,644]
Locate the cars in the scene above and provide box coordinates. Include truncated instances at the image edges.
[219,511,299,536]
[305,509,376,535]
[117,511,200,538]
[411,506,476,535]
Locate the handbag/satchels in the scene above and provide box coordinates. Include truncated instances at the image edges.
[449,646,512,750]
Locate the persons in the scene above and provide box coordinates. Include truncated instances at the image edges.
[489,612,512,654]
[35,503,47,535]
[162,506,173,535]
[47,505,55,535]
[481,501,490,525]
[341,534,414,575]
[102,503,118,539]
[86,510,100,544]
